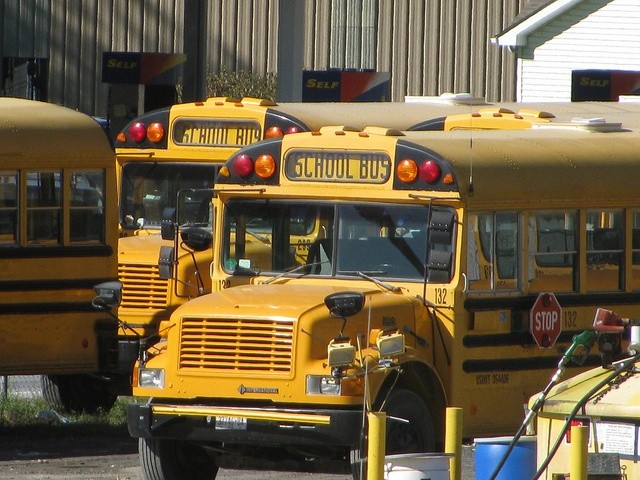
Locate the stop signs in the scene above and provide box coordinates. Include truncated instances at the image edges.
[528,292,562,349]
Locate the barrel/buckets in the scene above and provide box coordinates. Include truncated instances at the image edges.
[474,435,538,479]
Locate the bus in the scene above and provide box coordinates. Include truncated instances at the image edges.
[126,117,640,480]
[527,308,639,479]
[43,92,513,413]
[0,98,122,380]
